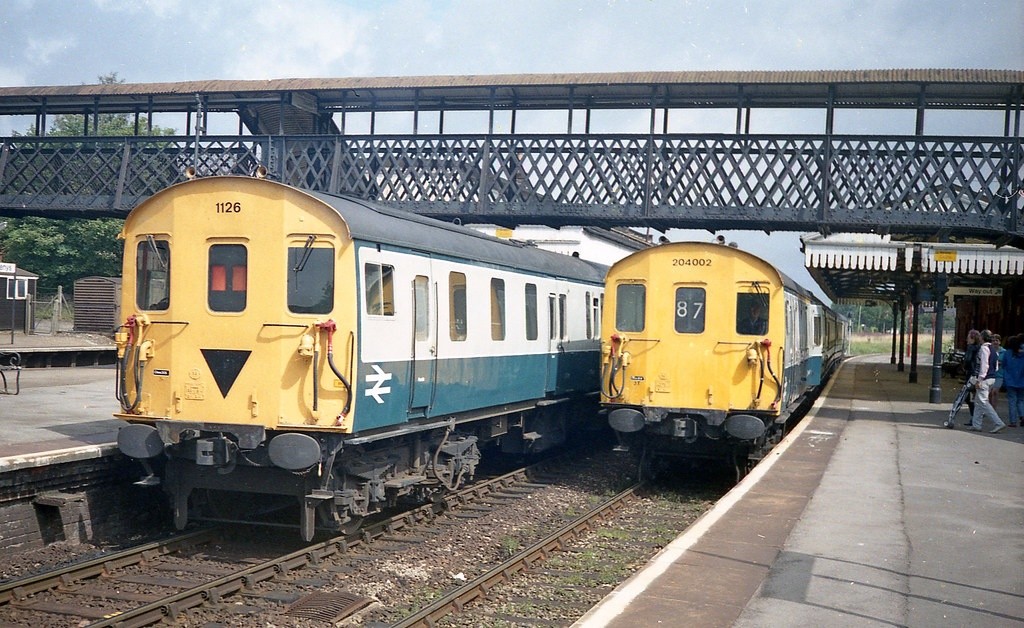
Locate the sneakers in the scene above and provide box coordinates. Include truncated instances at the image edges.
[1020,419,1024,425]
[989,425,1005,433]
[967,426,982,431]
[964,418,973,426]
[1008,421,1017,427]
[943,421,954,427]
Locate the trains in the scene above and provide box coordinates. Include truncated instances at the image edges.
[596,240,855,493]
[106,170,615,543]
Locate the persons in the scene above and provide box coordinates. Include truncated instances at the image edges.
[942,329,1024,433]
[736,302,767,335]
